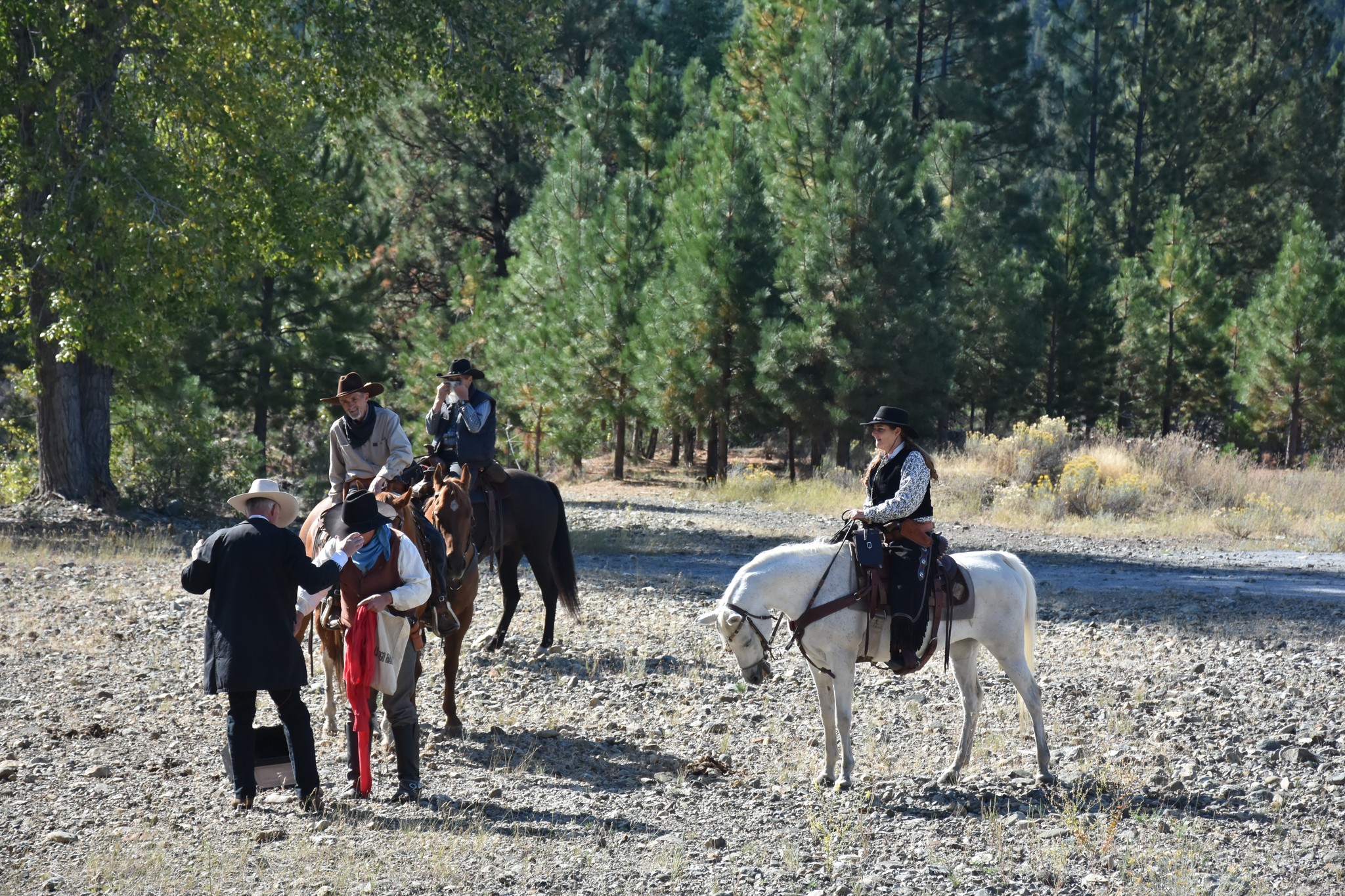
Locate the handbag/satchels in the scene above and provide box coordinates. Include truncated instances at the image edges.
[344,607,410,697]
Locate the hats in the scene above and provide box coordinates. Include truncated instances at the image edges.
[859,406,920,440]
[320,372,385,406]
[435,359,485,380]
[323,489,398,541]
[227,479,300,528]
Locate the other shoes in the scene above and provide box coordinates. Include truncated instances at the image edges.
[231,797,253,809]
[427,603,459,632]
[900,649,917,669]
[391,779,424,805]
[299,794,325,812]
[340,779,363,800]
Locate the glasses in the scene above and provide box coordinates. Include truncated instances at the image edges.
[448,377,466,382]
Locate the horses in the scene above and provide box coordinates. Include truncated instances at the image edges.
[297,478,429,754]
[420,461,480,742]
[692,532,1060,795]
[400,457,587,659]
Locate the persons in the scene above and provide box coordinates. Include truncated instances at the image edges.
[292,493,431,800]
[848,405,938,671]
[425,359,497,495]
[319,372,462,634]
[181,479,364,811]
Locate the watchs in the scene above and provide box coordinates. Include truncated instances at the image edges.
[459,399,470,406]
